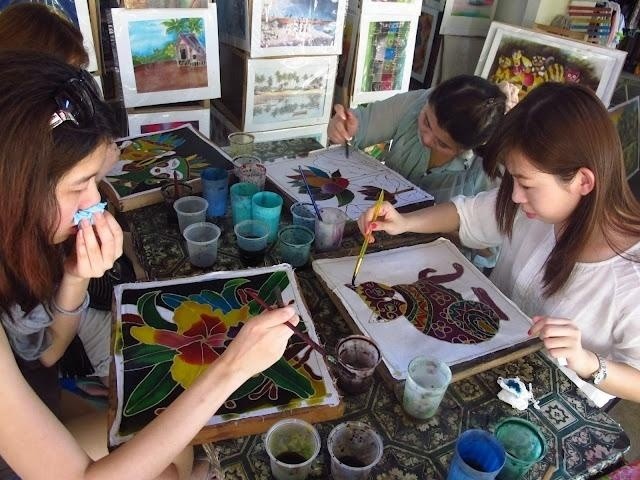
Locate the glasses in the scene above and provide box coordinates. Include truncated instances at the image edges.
[49,68,104,131]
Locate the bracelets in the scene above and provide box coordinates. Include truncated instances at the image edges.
[52,290,90,316]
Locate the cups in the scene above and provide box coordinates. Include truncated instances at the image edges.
[327,421,383,480]
[227,132,256,160]
[495,417,548,480]
[448,428,507,480]
[265,418,322,480]
[231,155,262,183]
[402,356,452,420]
[334,335,384,393]
[172,161,348,269]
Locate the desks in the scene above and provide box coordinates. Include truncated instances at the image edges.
[116,135,631,479]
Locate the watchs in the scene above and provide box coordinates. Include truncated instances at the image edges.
[576,352,608,385]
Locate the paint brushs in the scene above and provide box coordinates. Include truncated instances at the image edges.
[352,188,384,285]
[298,165,323,222]
[245,290,356,379]
[343,87,349,159]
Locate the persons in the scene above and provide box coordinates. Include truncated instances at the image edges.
[0,49,301,480]
[327,75,506,269]
[356,81,640,410]
[0,1,148,389]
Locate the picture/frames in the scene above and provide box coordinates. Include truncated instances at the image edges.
[211,43,339,146]
[412,0,496,89]
[336,0,424,110]
[127,109,210,140]
[110,2,221,108]
[29,1,105,101]
[216,2,348,58]
[477,27,620,112]
